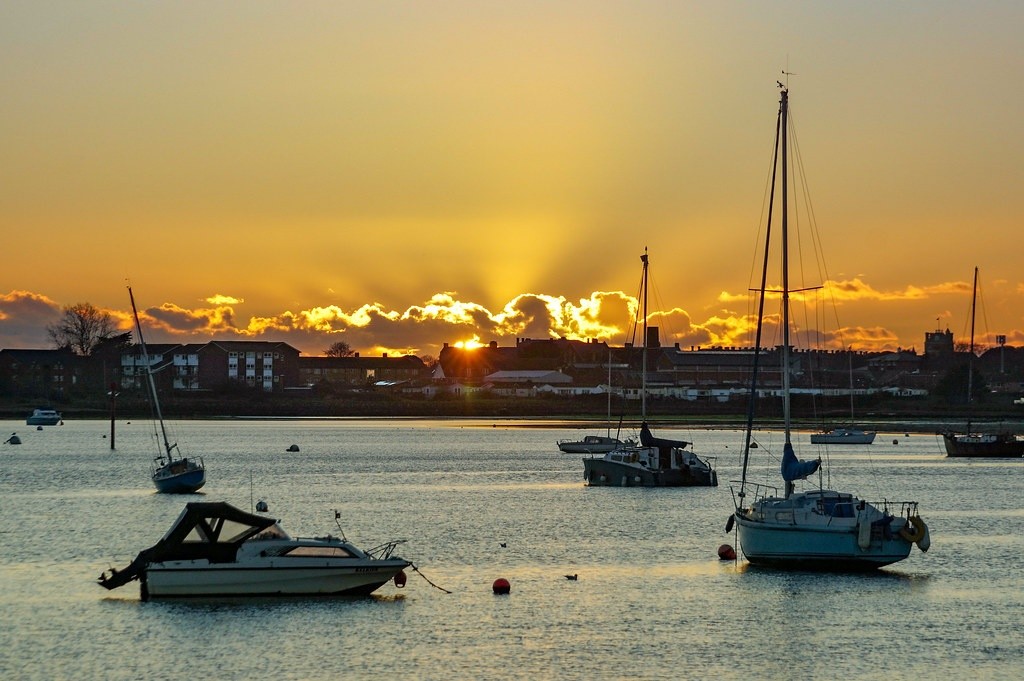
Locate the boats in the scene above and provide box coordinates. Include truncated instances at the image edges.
[96,467,413,607]
[25,407,64,427]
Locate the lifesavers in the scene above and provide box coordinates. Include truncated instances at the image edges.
[898,516,926,543]
[630,452,640,463]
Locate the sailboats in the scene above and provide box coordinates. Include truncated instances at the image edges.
[556,349,640,454]
[935,266,1024,458]
[727,69,934,575]
[581,246,720,487]
[123,277,208,496]
[810,346,878,445]
